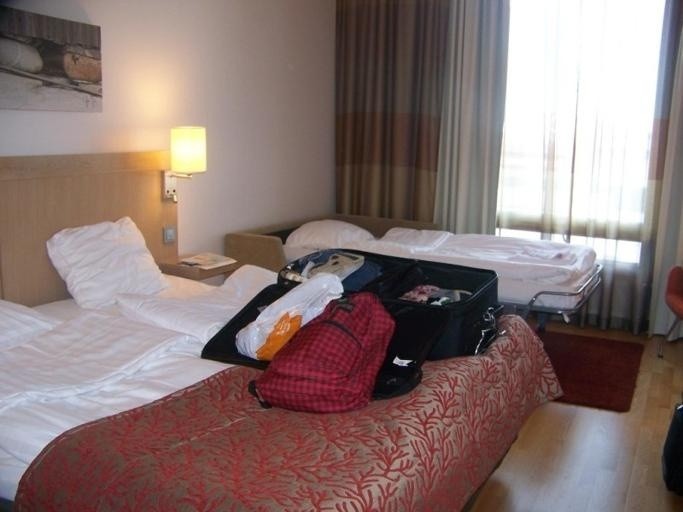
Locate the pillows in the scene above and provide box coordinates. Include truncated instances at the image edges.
[45,215,169,309]
[0,300,61,350]
[286,219,372,251]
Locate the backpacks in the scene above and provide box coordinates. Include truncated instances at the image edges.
[248,292,394,413]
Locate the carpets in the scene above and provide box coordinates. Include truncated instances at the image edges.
[538,328,644,412]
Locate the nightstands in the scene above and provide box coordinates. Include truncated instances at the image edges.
[160,253,238,285]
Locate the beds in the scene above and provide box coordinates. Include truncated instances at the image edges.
[225,212,604,338]
[1,146,564,512]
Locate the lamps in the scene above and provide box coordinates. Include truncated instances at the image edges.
[162,125,207,202]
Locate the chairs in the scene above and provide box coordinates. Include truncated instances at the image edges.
[659,267,682,357]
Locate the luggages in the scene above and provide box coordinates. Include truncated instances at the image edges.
[201,248,504,399]
